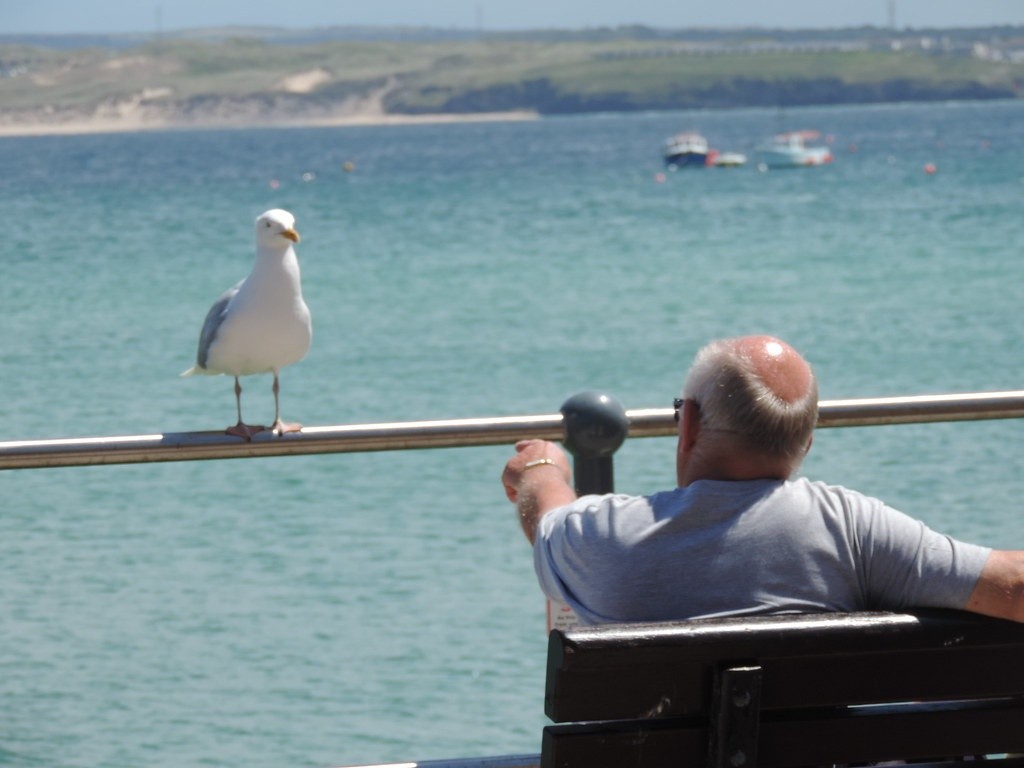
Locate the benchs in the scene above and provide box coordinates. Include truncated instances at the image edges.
[540,607,1024,768]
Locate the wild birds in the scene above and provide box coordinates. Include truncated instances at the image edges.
[179,208,312,443]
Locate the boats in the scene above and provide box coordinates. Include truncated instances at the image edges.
[660,133,707,163]
[712,153,746,167]
[760,135,834,169]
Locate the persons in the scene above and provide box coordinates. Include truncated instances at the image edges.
[502,335,1024,768]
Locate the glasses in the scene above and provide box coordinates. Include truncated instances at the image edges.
[673,398,701,421]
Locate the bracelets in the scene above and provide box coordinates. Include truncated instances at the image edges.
[518,459,560,472]
